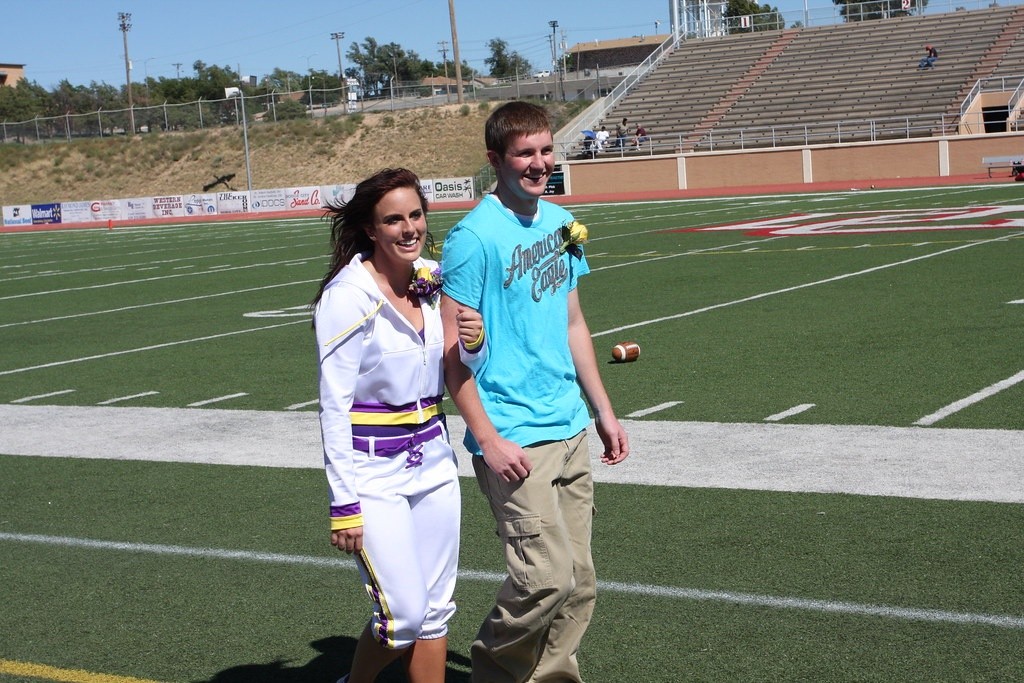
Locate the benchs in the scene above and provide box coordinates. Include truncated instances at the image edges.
[980,155,1024,178]
[570,4,1024,157]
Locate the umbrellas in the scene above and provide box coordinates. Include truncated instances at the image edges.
[580,130,597,140]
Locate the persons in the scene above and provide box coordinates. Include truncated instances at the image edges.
[440,101,629,683]
[632,124,648,151]
[307,168,488,683]
[584,126,610,159]
[919,45,938,69]
[615,118,630,151]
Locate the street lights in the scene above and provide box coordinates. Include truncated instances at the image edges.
[117,11,135,135]
[143,57,156,122]
[298,53,319,110]
[548,20,559,102]
[331,31,347,114]
[224,87,251,191]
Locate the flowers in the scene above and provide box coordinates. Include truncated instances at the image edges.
[408,266,445,310]
[558,218,588,262]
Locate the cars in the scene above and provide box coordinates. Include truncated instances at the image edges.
[533,69,551,79]
